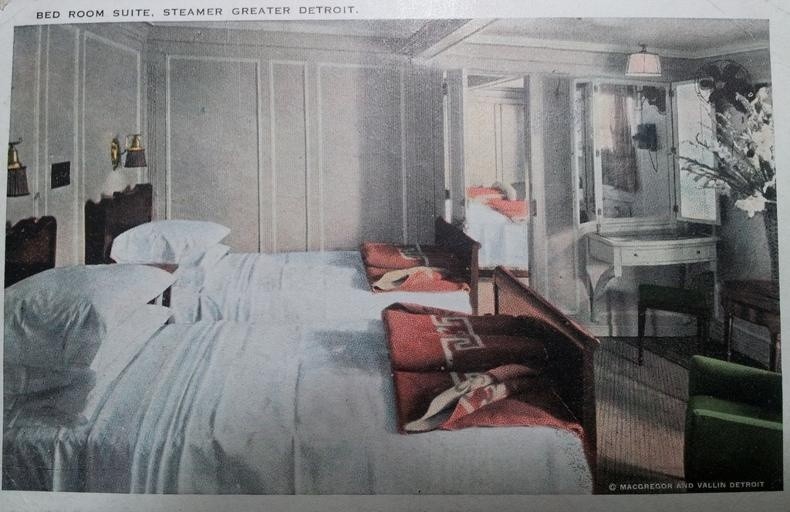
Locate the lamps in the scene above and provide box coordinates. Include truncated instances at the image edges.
[625,43,664,77]
[110,130,146,172]
[8,137,31,197]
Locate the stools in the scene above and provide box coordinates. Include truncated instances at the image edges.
[636,270,716,368]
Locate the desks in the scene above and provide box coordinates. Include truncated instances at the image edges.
[584,229,723,321]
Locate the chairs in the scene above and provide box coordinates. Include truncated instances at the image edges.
[683,355,782,491]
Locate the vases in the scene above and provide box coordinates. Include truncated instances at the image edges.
[763,200,781,297]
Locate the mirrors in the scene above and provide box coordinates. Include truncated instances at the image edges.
[573,78,724,228]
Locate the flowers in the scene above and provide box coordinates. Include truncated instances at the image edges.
[671,87,779,219]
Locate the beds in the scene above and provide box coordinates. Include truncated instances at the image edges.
[6,186,602,494]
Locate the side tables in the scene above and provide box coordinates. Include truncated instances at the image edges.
[719,279,781,370]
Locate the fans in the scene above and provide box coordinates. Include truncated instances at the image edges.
[693,59,753,116]
